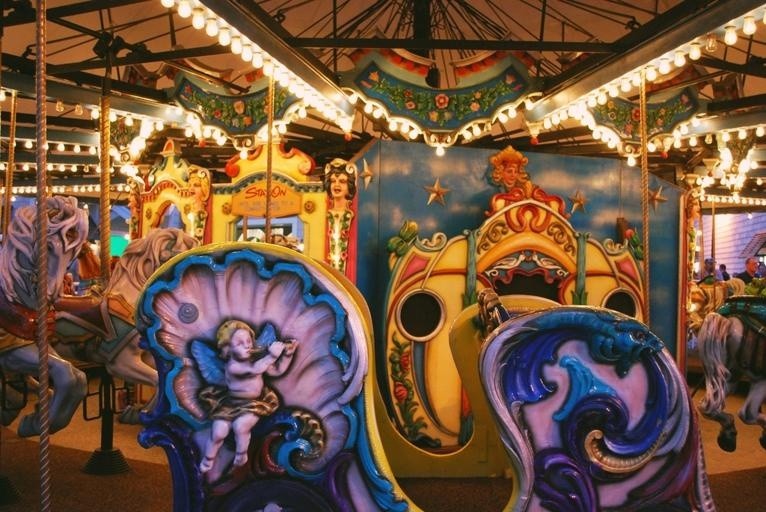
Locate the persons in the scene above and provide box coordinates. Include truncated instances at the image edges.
[197,317,297,474]
[488,146,530,191]
[73,239,121,298]
[694,256,766,286]
[324,158,358,210]
